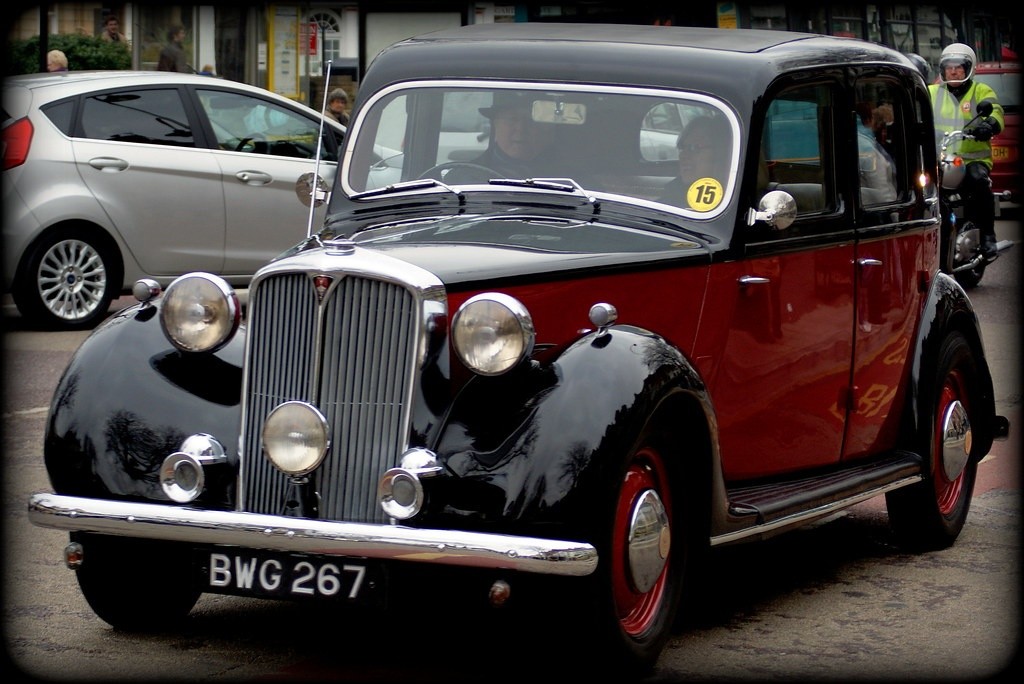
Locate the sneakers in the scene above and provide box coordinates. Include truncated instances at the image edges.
[981,235,998,262]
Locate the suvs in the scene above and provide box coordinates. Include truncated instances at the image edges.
[23,20,1011,684]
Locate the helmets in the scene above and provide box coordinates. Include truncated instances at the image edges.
[907,53,930,83]
[938,43,976,83]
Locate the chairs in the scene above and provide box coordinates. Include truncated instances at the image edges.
[632,176,885,212]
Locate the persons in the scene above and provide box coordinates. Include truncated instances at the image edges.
[855,102,898,205]
[46,50,68,72]
[927,43,1004,259]
[660,115,795,210]
[322,87,351,126]
[158,25,188,73]
[102,17,127,43]
[443,92,589,190]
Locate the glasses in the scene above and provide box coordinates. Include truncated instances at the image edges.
[678,143,715,153]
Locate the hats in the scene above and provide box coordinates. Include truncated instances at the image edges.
[478,91,533,119]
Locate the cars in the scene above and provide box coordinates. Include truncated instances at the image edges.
[2,69,449,332]
[372,86,893,193]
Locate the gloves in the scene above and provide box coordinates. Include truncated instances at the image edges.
[971,116,1002,142]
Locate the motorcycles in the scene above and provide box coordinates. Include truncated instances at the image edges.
[926,83,1022,293]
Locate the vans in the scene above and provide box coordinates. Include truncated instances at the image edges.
[932,59,1024,203]
